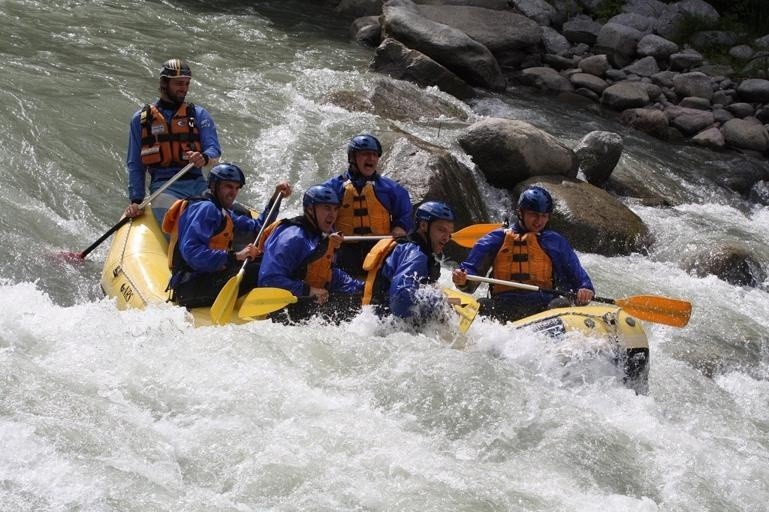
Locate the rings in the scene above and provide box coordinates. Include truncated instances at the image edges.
[587,299,591,303]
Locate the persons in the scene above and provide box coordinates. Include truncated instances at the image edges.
[124,58,221,242]
[255,183,367,326]
[162,161,291,309]
[320,134,414,277]
[372,201,455,332]
[452,186,594,324]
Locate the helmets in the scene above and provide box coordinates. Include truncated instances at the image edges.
[516,185,553,214]
[303,184,340,207]
[347,133,382,165]
[206,162,246,189]
[159,58,192,80]
[413,200,455,227]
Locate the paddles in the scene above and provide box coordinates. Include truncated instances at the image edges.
[203,189,283,327]
[442,294,482,333]
[64,161,197,263]
[344,222,502,249]
[460,272,692,328]
[238,282,314,328]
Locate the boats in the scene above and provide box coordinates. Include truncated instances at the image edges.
[101,202,649,403]
